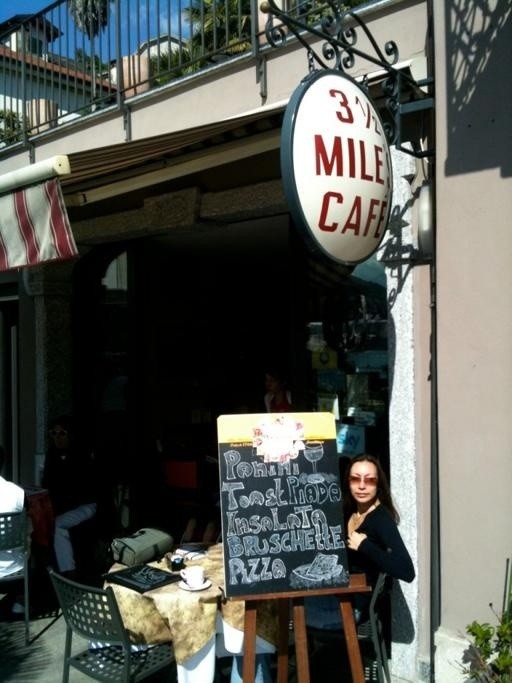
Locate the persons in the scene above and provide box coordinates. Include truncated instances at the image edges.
[4,359,315,617]
[226,449,418,682]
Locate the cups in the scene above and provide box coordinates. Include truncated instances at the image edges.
[179,566,204,587]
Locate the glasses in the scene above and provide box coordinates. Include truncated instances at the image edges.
[349,475,378,487]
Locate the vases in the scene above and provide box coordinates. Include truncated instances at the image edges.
[123,56,155,96]
[28,98,58,136]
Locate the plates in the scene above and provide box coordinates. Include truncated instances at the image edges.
[178,579,212,592]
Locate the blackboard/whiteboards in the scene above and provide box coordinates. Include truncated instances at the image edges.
[212,411,353,598]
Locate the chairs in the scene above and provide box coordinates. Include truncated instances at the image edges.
[306,572,391,683]
[0,508,30,648]
[45,566,176,683]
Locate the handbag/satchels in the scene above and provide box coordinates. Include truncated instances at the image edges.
[109,525,175,568]
